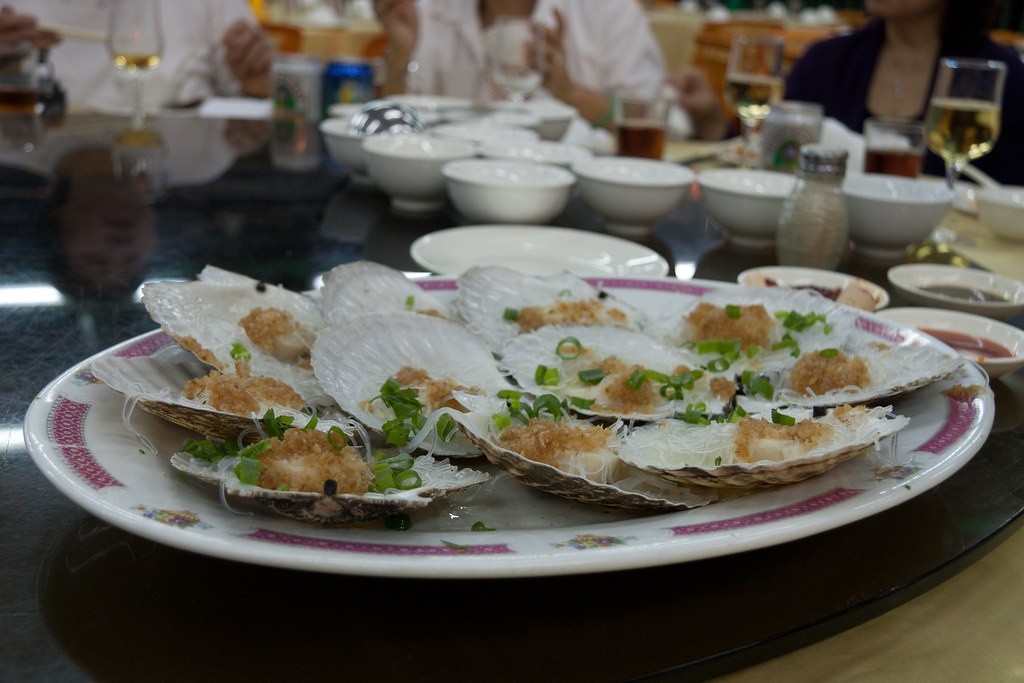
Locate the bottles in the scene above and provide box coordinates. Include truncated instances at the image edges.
[775,143,850,272]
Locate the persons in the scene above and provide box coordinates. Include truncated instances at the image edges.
[0,110,278,302]
[370,0,692,140]
[782,1,1024,190]
[0,1,276,106]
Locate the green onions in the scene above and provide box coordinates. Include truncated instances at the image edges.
[179,291,839,529]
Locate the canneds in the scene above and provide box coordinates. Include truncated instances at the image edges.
[267,53,373,174]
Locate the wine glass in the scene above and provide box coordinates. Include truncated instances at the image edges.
[715,34,784,168]
[924,57,1005,194]
[106,0,165,151]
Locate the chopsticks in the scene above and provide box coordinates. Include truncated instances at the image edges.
[36,16,137,46]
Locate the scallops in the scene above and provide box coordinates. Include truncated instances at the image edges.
[85,350,361,446]
[664,292,968,405]
[310,306,516,456]
[167,441,486,521]
[451,412,725,515]
[618,405,912,491]
[314,259,456,319]
[467,264,644,350]
[506,323,737,425]
[138,272,322,381]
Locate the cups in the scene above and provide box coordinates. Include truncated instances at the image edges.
[612,91,672,162]
[862,114,928,179]
[481,12,546,102]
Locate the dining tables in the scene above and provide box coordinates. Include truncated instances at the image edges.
[0,111,1024,682]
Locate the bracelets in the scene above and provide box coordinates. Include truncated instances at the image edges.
[595,94,614,128]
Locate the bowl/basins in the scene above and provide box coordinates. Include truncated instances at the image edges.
[329,88,1023,260]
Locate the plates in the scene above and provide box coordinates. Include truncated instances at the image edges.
[738,265,889,312]
[410,222,669,278]
[875,306,1024,379]
[24,275,996,582]
[886,261,1023,320]
